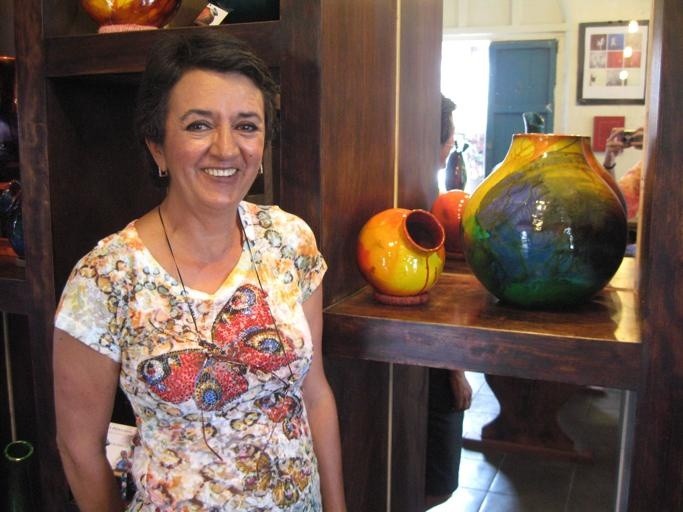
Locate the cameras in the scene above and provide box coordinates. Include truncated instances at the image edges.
[614,128,642,145]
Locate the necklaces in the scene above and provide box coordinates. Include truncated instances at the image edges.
[154,204,295,382]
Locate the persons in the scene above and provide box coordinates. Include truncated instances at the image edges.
[54,30,347,512]
[600,123,649,223]
[423,88,472,510]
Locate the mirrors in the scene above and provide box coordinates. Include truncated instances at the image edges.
[383,355,632,512]
[388,0,654,290]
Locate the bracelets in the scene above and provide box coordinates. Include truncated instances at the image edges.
[601,164,616,169]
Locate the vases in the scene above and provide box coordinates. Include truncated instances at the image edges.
[426,188,473,264]
[456,133,632,315]
[0,441,44,512]
[350,205,450,308]
[80,0,182,33]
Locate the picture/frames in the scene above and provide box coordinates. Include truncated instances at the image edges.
[574,19,649,106]
